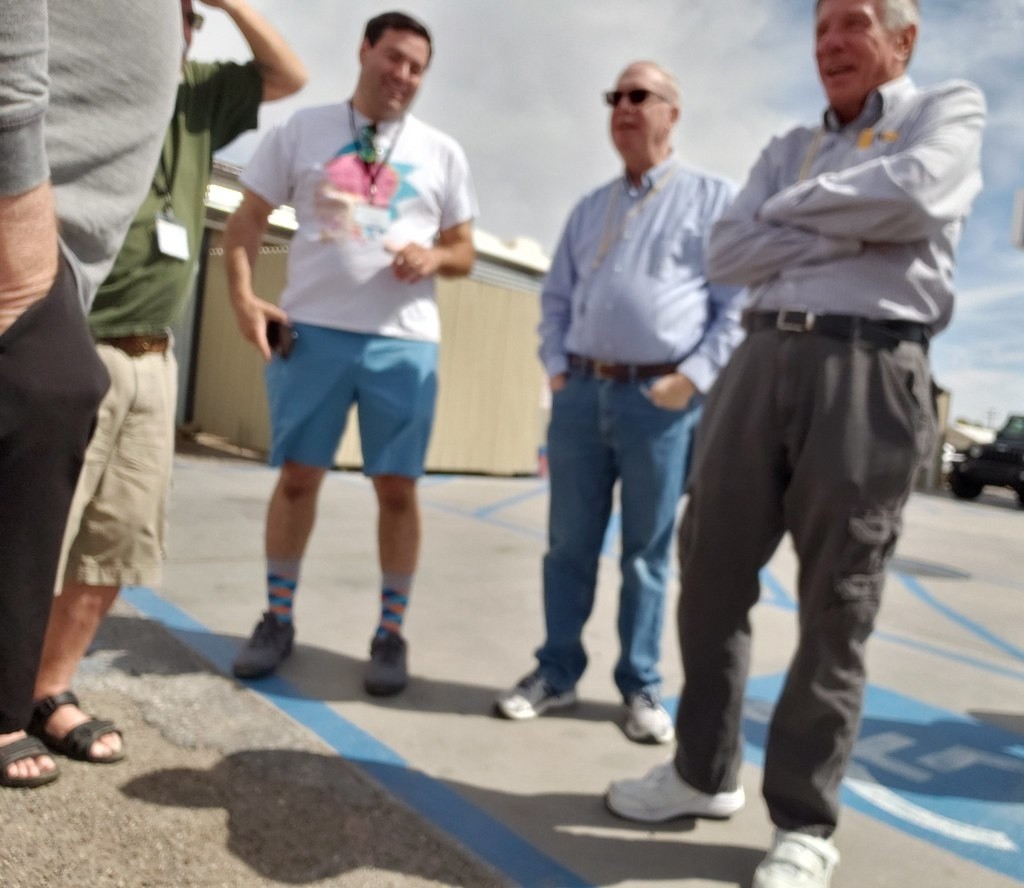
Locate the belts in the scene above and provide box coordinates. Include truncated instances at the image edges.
[92,336,170,357]
[567,351,679,382]
[739,308,930,347]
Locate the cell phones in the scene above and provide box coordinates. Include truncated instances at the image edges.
[266,320,298,359]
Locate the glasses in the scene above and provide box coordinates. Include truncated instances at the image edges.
[357,121,377,174]
[605,88,668,104]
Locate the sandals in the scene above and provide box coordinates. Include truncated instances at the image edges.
[0,692,126,788]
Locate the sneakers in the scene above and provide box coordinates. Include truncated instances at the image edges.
[620,694,675,746]
[364,637,408,697]
[604,759,747,824]
[233,612,295,680]
[496,672,578,718]
[752,828,839,888]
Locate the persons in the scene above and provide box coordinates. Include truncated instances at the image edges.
[1,0,308,792]
[224,8,484,694]
[1,2,187,740]
[496,61,752,748]
[604,2,990,888]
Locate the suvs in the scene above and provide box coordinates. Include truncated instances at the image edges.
[942,414,1024,509]
